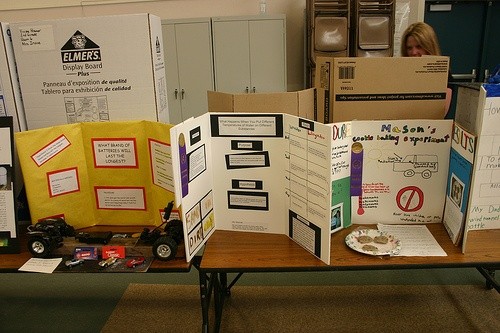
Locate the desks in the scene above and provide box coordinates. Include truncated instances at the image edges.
[199,224,500,333]
[0,225,213,333]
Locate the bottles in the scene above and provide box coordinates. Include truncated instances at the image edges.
[488,74,494,83]
[484,70,489,83]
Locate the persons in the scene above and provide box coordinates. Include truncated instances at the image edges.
[402,22,454,119]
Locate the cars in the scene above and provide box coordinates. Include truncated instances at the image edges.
[65,258,85,268]
[125,256,147,268]
[97,256,118,268]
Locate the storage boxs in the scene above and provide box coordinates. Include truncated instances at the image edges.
[8,13,170,131]
[0,22,27,198]
[314,54,450,124]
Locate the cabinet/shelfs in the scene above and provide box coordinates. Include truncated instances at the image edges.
[211,14,288,94]
[160,17,215,125]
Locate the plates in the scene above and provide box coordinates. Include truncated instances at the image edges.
[345,229,402,255]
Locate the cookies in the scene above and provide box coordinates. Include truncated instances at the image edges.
[358,235,389,251]
